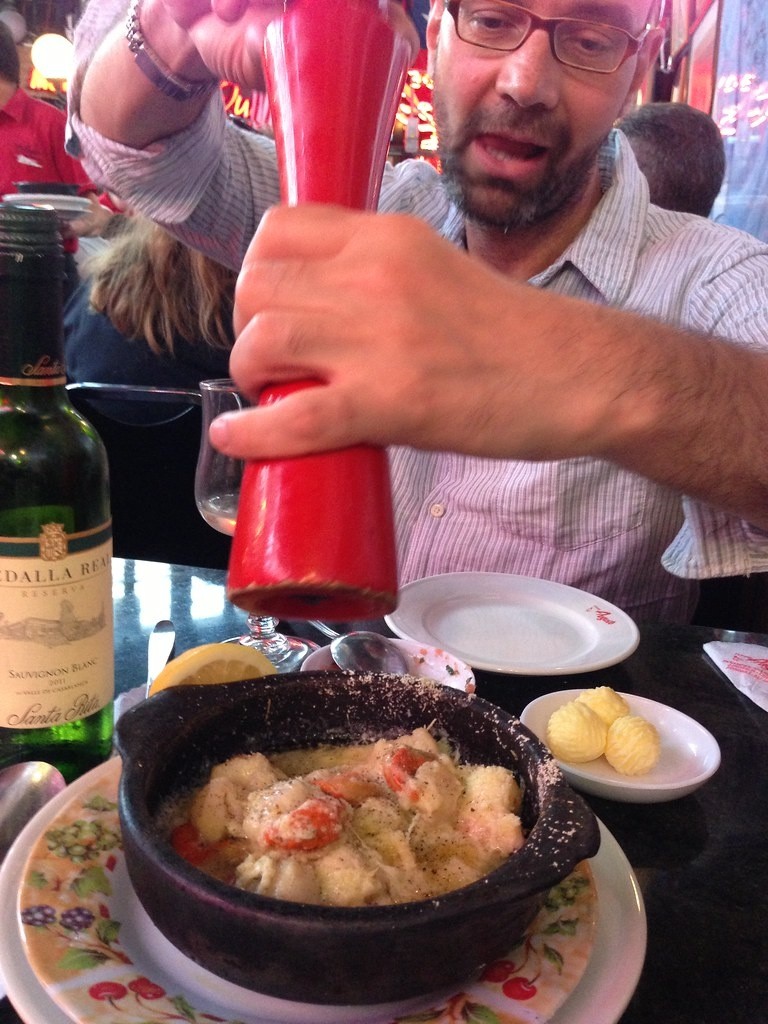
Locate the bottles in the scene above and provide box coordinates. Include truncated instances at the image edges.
[0,207,113,790]
[222,2,412,623]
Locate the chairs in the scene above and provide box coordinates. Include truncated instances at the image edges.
[69,382,232,573]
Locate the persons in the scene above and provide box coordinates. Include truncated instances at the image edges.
[58,207,240,397]
[97,189,128,215]
[610,101,727,220]
[0,21,114,251]
[61,0,768,636]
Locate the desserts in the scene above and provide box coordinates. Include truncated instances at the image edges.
[548,684,662,776]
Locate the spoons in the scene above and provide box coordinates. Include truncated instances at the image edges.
[306,619,409,677]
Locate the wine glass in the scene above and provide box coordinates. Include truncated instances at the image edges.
[196,380,319,674]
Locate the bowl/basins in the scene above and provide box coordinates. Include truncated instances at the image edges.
[13,182,80,195]
[299,639,477,694]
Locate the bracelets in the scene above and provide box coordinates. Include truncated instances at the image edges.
[127,0,220,100]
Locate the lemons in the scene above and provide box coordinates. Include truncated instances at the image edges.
[148,643,276,700]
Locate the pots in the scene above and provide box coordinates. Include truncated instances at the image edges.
[111,669,601,1007]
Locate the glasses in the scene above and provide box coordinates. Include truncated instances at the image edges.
[446,0,651,74]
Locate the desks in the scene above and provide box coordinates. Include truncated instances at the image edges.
[115,553,768,1024]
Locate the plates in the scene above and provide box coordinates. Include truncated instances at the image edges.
[384,571,641,676]
[0,753,649,1023]
[3,194,92,209]
[521,689,722,804]
[55,210,93,221]
